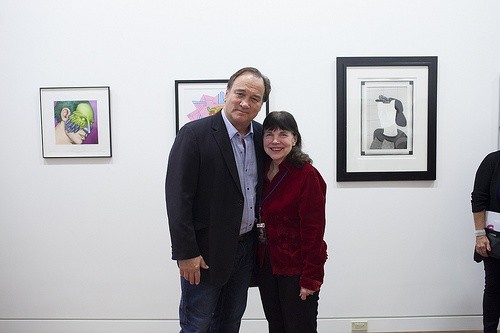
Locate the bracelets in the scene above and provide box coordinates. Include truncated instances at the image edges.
[475,229,486,236]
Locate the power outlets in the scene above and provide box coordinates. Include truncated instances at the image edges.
[352,321,368,331]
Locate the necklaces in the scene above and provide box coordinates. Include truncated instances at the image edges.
[256,169,288,241]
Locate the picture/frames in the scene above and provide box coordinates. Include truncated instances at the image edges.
[39,87,113,158]
[335,55,437,181]
[175,80,270,139]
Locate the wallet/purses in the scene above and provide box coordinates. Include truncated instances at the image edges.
[485,233,500,257]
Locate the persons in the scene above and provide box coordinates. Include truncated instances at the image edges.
[258,111,328,333]
[165,67,273,333]
[471,150,500,333]
